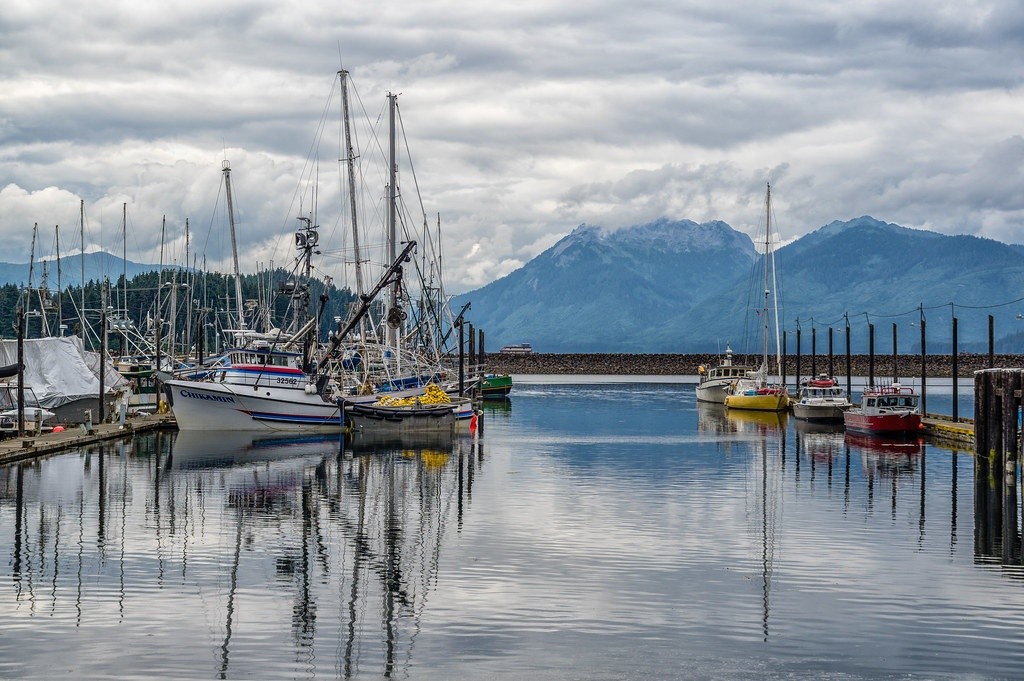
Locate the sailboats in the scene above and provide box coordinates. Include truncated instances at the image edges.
[0,41,513,433]
[721,182,792,412]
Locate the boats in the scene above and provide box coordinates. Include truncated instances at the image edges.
[695,341,753,404]
[499,342,532,353]
[793,373,854,419]
[841,375,924,431]
